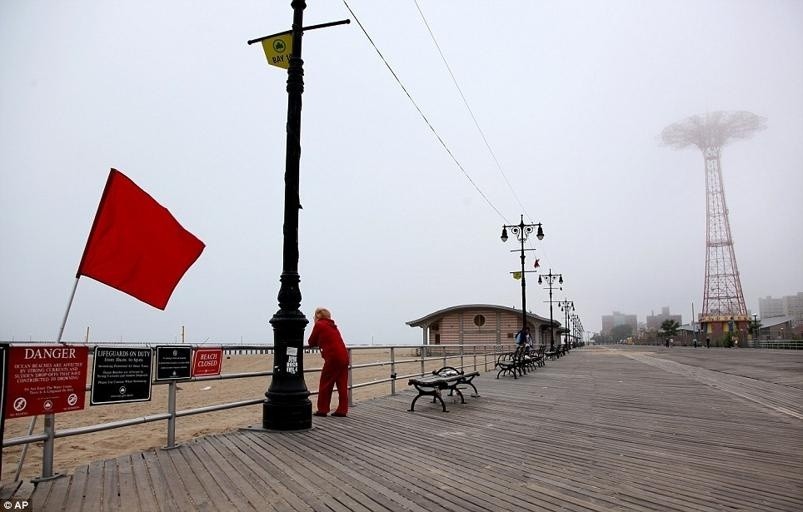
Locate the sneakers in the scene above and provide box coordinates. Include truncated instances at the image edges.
[313,411,326,417]
[331,411,345,416]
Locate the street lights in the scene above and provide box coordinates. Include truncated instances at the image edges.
[752,313,758,338]
[536,267,562,353]
[558,295,602,350]
[501,213,545,340]
[780,327,784,338]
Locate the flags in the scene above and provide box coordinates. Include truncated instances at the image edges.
[76,168,206,310]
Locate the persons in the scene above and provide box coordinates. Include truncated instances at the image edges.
[666,336,710,349]
[515,327,533,360]
[308,309,350,417]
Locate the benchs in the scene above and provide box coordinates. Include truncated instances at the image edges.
[406,366,480,412]
[495,348,569,380]
